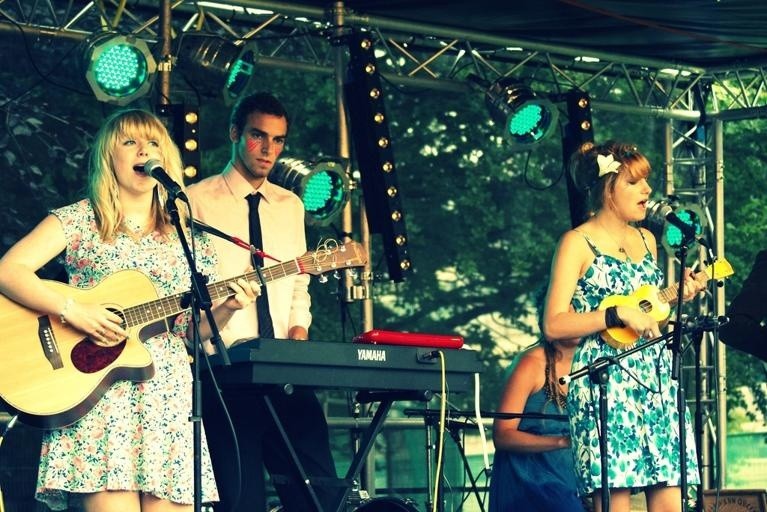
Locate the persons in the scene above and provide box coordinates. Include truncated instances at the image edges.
[489,286,593,512]
[0,107,262,512]
[542,139,703,510]
[186,93,347,512]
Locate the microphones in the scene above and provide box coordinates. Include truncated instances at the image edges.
[681,313,728,326]
[145,158,187,204]
[646,197,713,252]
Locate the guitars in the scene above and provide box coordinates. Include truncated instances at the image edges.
[596,258,735,349]
[0,239,369,431]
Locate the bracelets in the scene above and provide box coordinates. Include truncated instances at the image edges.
[59,298,77,323]
[606,306,627,329]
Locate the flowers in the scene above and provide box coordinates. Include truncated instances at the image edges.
[595,153,622,178]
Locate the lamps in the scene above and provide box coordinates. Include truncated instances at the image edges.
[171,30,258,107]
[466,73,560,150]
[76,30,158,108]
[634,194,708,255]
[267,152,353,228]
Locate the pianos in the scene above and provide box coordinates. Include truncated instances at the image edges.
[191,338,483,394]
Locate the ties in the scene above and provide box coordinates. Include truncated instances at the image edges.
[245,192,274,338]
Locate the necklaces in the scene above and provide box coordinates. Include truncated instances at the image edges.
[595,215,628,253]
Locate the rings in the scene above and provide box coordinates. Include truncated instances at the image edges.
[99,328,108,336]
